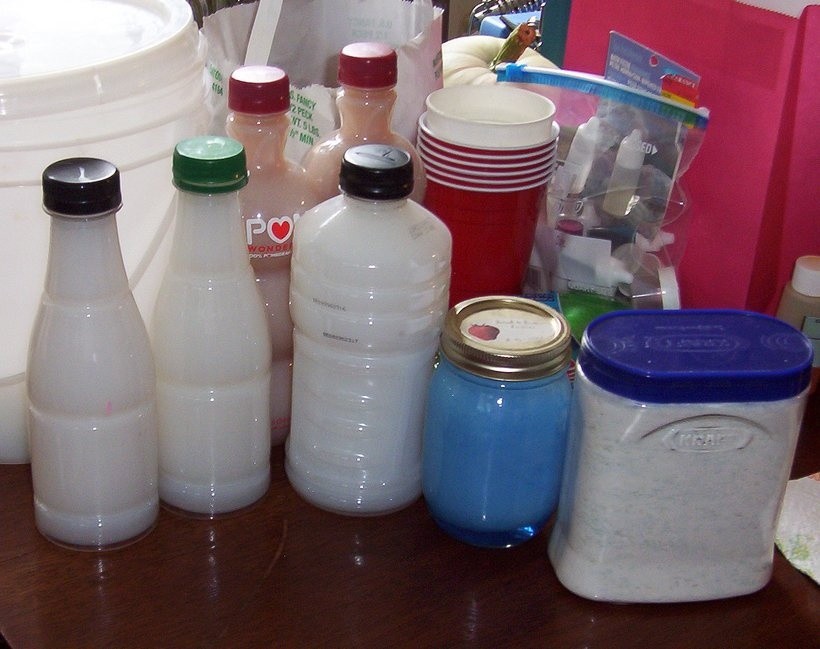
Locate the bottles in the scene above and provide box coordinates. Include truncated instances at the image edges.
[601,129,645,217]
[775,256,820,397]
[419,293,574,548]
[24,155,160,556]
[547,308,815,603]
[561,116,600,194]
[151,134,273,518]
[297,39,424,210]
[224,65,318,444]
[285,143,454,513]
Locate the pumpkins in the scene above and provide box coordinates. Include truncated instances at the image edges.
[441,22,560,86]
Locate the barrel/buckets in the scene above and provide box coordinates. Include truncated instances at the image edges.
[0,1,218,463]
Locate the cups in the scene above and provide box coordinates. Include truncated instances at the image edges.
[417,84,562,306]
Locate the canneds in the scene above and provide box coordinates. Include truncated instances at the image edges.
[419,295,573,546]
